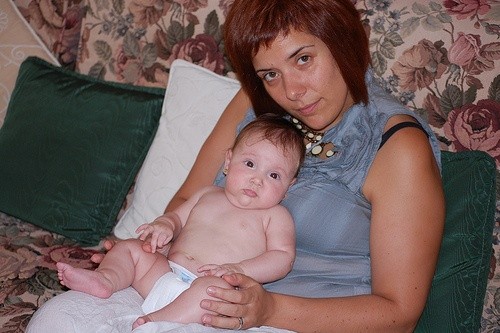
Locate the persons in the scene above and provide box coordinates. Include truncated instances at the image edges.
[26,0,445,333]
[56,113,306,330]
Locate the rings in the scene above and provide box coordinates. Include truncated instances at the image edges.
[237,317,243,329]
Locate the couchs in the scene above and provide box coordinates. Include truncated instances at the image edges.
[0,0,500,333]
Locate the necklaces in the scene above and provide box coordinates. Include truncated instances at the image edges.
[290,116,338,159]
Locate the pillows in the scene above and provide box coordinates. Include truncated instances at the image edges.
[413,149,496,333]
[113,58,242,241]
[0,0,65,129]
[0,55,166,246]
